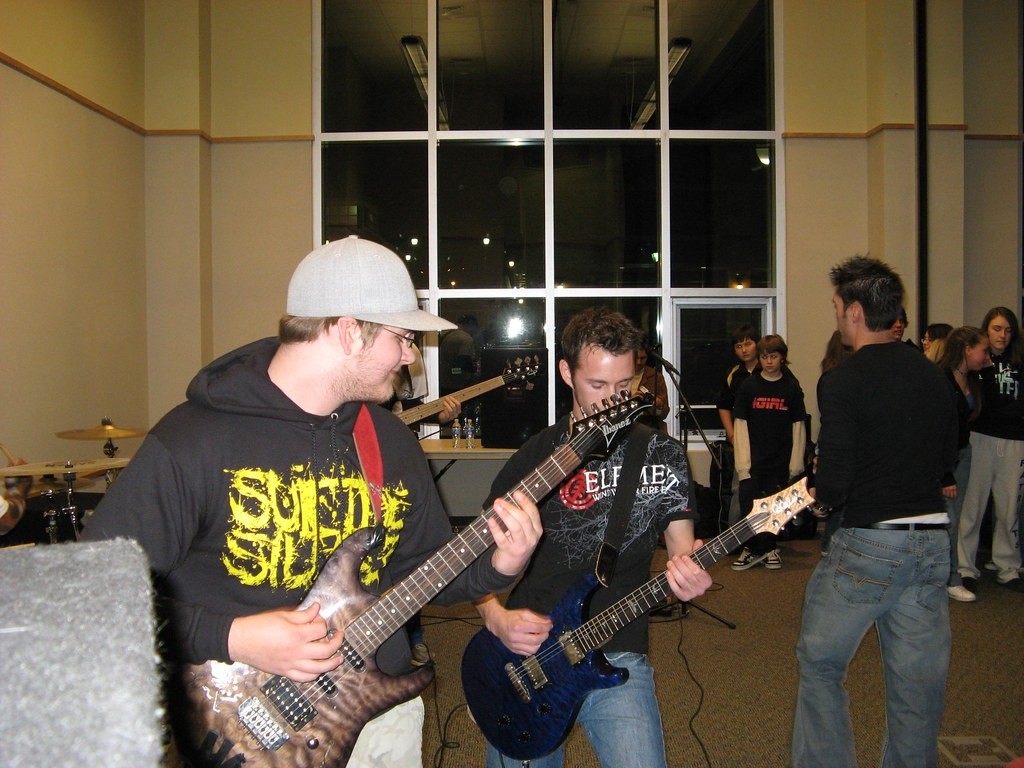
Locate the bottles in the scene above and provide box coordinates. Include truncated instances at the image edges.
[465,419,475,449]
[451,419,462,448]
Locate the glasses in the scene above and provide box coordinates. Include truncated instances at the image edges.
[383,327,416,348]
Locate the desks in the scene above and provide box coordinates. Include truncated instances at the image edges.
[420,438,519,518]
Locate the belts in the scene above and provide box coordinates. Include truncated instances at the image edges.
[846,522,951,530]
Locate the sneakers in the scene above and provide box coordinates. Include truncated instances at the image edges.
[760,550,783,569]
[731,546,767,570]
[947,585,976,601]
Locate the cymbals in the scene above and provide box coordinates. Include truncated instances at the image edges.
[55,422,149,441]
[0,457,134,478]
[22,481,97,500]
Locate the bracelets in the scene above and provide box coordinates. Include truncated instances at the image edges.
[728,435,735,442]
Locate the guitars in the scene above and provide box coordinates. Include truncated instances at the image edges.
[162,389,662,768]
[391,355,541,425]
[460,474,817,761]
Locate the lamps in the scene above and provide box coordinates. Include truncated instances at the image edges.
[398,0,450,131]
[630,0,693,131]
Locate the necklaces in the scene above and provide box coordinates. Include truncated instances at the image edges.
[957,368,968,376]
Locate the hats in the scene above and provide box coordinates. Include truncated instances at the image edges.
[287,234,458,332]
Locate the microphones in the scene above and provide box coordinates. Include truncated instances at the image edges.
[646,347,678,373]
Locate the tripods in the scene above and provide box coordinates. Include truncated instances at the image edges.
[649,364,736,629]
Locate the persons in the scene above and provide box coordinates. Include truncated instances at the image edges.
[378,343,461,439]
[717,323,806,571]
[629,346,670,549]
[791,251,970,768]
[0,458,34,535]
[821,307,1024,601]
[440,314,477,367]
[80,236,544,768]
[473,307,713,768]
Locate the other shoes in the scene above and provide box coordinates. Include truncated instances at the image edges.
[961,576,978,592]
[997,578,1024,592]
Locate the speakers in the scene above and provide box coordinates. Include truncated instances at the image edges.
[481,345,548,449]
